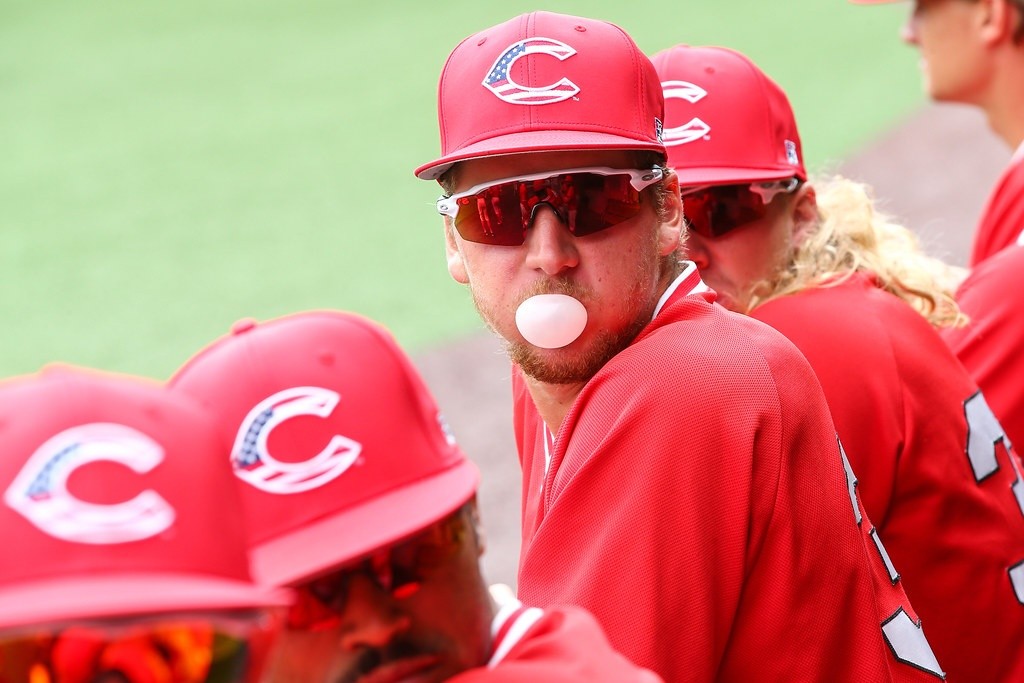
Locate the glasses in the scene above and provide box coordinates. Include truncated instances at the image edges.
[284,513,466,635]
[436,164,670,247]
[681,178,803,237]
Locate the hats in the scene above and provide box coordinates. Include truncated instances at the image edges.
[0,360,294,628]
[646,43,808,187]
[414,10,665,181]
[165,311,480,587]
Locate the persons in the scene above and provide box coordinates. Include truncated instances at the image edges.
[649,44,1023,683]
[0,366,268,682]
[906,0,1023,456]
[438,10,949,683]
[177,308,670,683]
